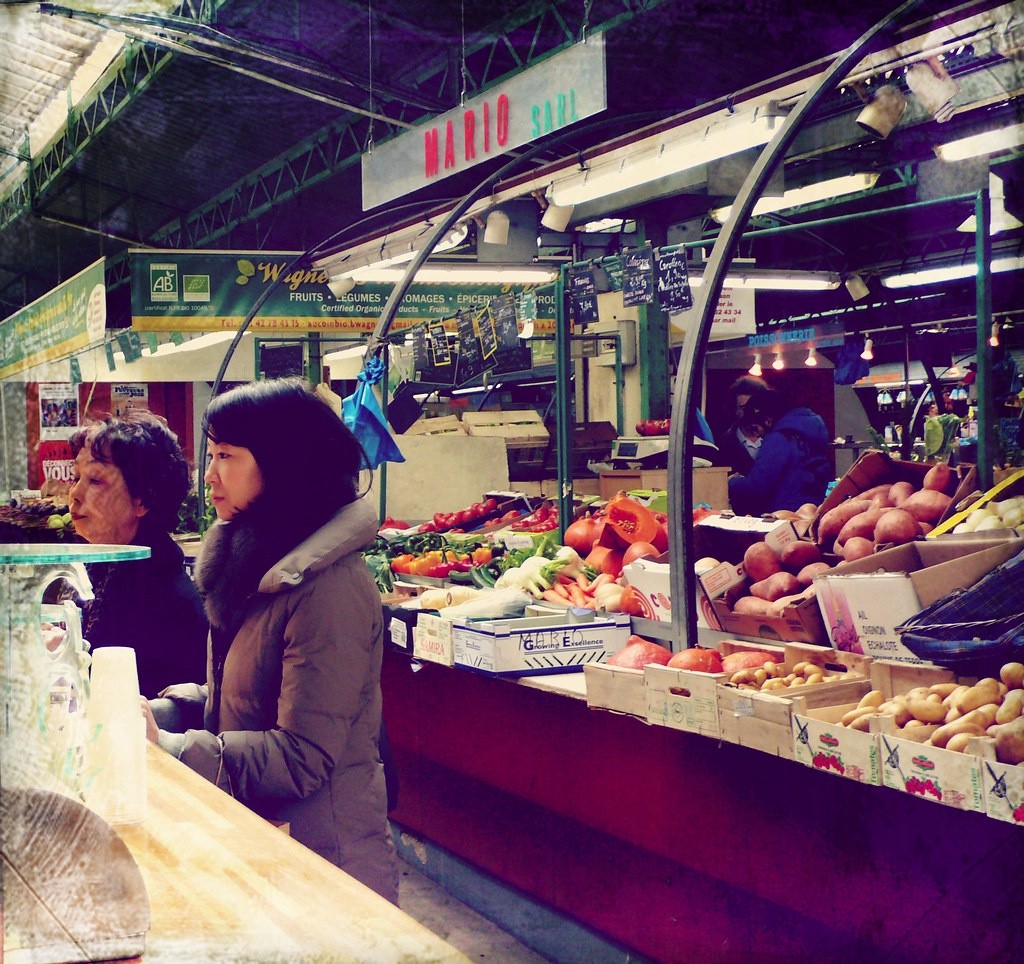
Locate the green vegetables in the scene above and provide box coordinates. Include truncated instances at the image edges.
[175,484,218,539]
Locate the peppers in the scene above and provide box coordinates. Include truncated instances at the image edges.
[371,532,496,579]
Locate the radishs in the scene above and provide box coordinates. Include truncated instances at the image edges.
[419,586,477,610]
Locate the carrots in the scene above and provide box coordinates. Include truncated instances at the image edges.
[541,564,625,609]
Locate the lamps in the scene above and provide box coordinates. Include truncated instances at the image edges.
[688,271,842,290]
[544,101,788,206]
[906,57,961,123]
[484,205,509,244]
[749,354,762,376]
[325,343,367,361]
[860,332,873,359]
[541,204,575,232]
[845,273,870,301]
[325,223,469,281]
[772,352,785,371]
[353,263,560,282]
[855,70,909,140]
[327,277,355,299]
[805,348,817,366]
[707,173,881,225]
[990,323,1000,346]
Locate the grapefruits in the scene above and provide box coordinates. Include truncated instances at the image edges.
[46,513,72,529]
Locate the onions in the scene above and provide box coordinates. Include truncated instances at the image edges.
[494,546,589,599]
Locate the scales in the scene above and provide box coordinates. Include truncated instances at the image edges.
[611,434,719,470]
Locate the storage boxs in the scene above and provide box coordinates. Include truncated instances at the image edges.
[378,489,1024,825]
[403,410,619,497]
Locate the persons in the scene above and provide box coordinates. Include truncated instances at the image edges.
[42,399,77,426]
[729,390,832,518]
[986,333,1023,418]
[57,409,209,699]
[957,362,977,386]
[115,400,135,416]
[709,376,771,478]
[137,376,401,909]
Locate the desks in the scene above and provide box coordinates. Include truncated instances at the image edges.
[377,636,1024,964]
[828,442,925,464]
[112,734,473,964]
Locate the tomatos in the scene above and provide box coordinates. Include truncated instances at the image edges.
[417,498,560,533]
[635,418,672,436]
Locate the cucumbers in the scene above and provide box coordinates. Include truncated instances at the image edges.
[448,564,497,588]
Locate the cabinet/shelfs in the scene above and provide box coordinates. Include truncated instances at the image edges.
[600,468,733,510]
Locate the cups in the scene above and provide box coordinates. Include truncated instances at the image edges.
[83,645,148,826]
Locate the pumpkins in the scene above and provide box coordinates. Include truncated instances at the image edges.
[563,490,722,577]
[594,584,645,616]
[603,634,776,696]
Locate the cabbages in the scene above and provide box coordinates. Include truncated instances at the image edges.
[441,586,533,620]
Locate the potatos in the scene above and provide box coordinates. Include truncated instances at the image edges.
[729,661,856,691]
[731,463,952,616]
[835,661,1024,771]
